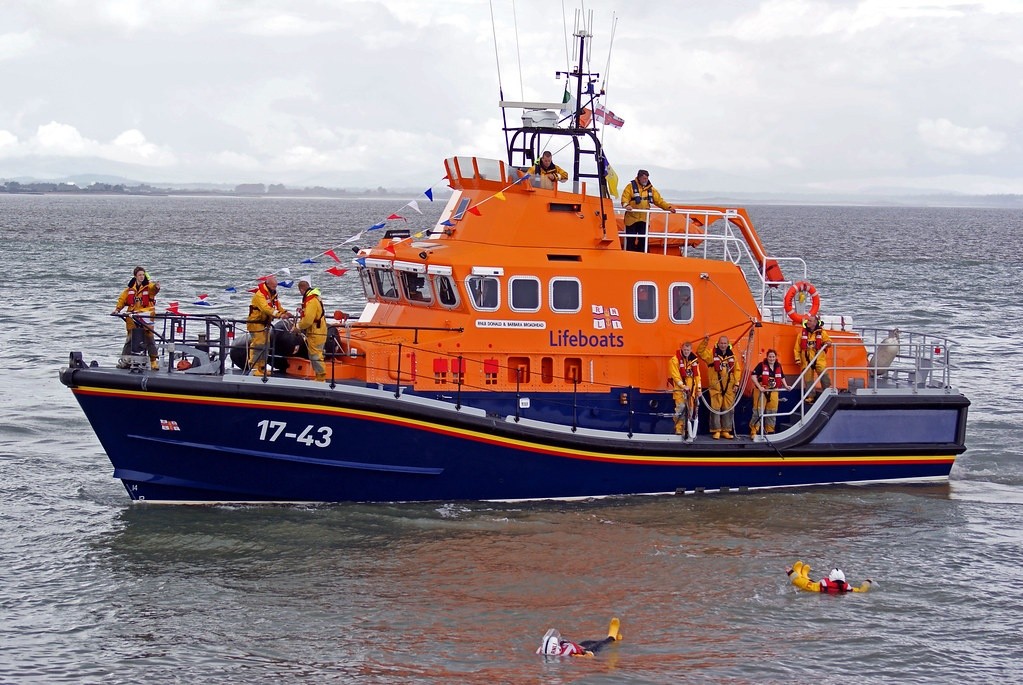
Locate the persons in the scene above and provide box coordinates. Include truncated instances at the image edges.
[748,350,792,439]
[111,267,161,370]
[669,342,702,435]
[247,277,294,376]
[638,286,653,319]
[621,169,676,253]
[534,617,623,659]
[290,281,327,384]
[696,333,741,439]
[516,151,568,190]
[784,561,872,595]
[794,316,833,401]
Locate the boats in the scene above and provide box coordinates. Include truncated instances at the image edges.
[58,29,971,504]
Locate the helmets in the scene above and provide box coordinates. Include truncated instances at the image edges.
[541,636,562,656]
[828,568,846,582]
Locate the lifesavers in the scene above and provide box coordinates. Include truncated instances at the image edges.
[783,281,821,324]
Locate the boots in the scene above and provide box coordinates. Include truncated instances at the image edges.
[793,561,811,580]
[750,431,757,439]
[607,618,623,641]
[675,424,683,434]
[251,362,273,376]
[805,397,814,402]
[713,432,734,439]
[151,361,160,369]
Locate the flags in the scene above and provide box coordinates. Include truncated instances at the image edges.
[589,103,625,131]
[560,86,593,129]
[598,148,619,200]
